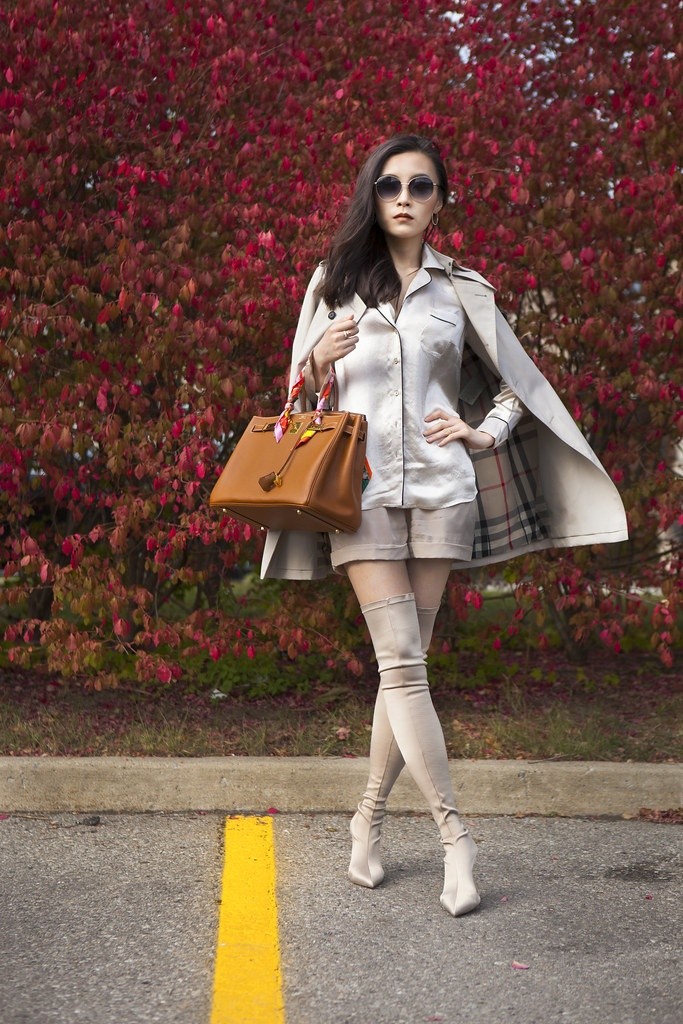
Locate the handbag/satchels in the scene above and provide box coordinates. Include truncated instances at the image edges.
[209,361,368,534]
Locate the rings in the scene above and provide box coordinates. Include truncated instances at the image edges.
[343,331,351,339]
[447,428,453,434]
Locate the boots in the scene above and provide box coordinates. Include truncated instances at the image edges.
[348,601,442,888]
[360,592,481,917]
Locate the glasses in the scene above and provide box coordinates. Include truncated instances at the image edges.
[373,173,442,204]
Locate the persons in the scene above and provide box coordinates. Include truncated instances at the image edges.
[260,136,629,917]
